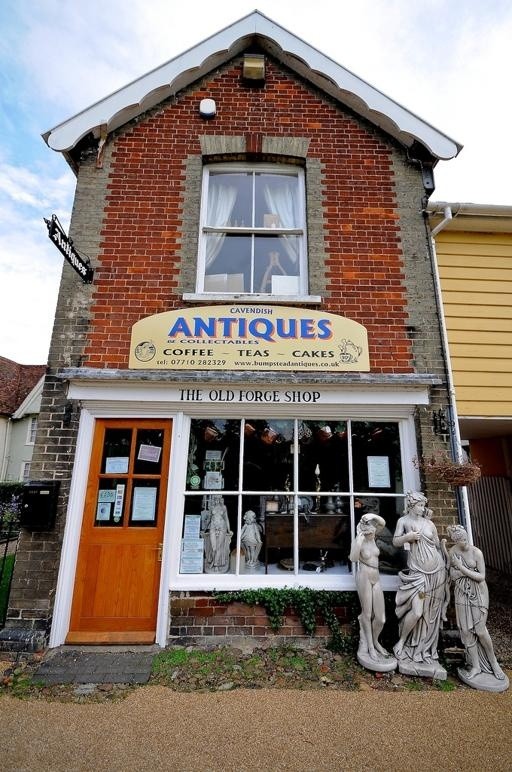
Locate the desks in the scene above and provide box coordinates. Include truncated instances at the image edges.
[259,512,359,574]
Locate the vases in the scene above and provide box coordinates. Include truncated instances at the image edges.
[443,466,481,486]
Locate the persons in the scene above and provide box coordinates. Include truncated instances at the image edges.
[238,510,263,571]
[349,513,391,658]
[393,491,447,662]
[208,495,234,567]
[442,523,504,680]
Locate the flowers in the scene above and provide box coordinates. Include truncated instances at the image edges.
[413,446,482,476]
